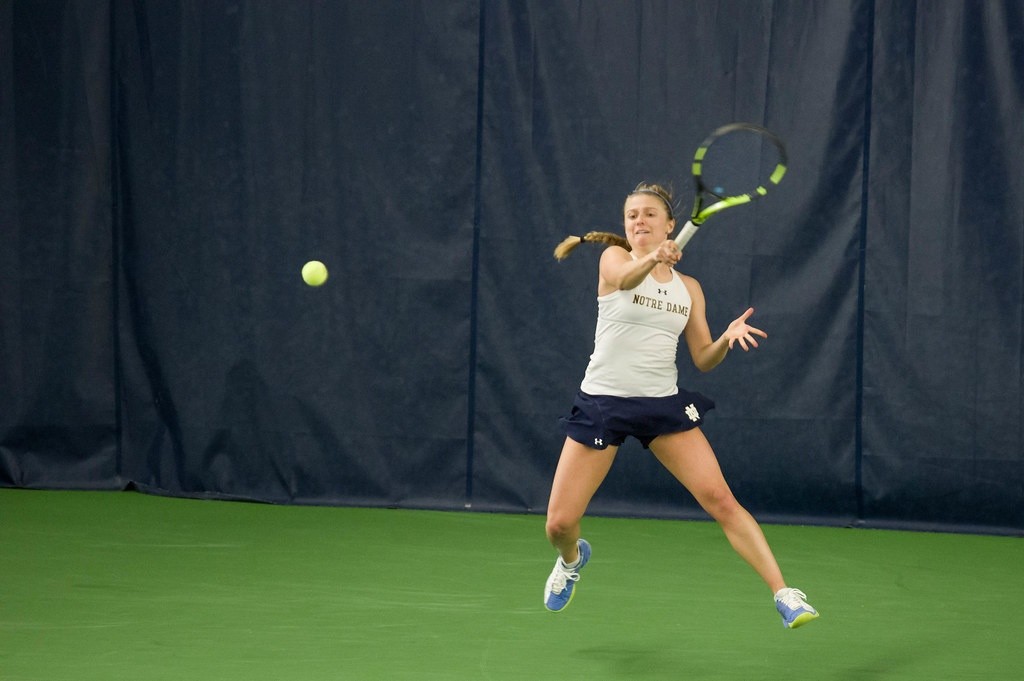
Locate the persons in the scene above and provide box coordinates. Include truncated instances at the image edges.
[543,180,821,629]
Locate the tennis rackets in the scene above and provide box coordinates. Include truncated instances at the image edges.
[670,121,788,258]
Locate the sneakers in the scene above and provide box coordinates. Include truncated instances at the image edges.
[544,539,592,612]
[775,587,819,629]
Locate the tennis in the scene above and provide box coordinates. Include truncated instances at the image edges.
[302,259,329,287]
